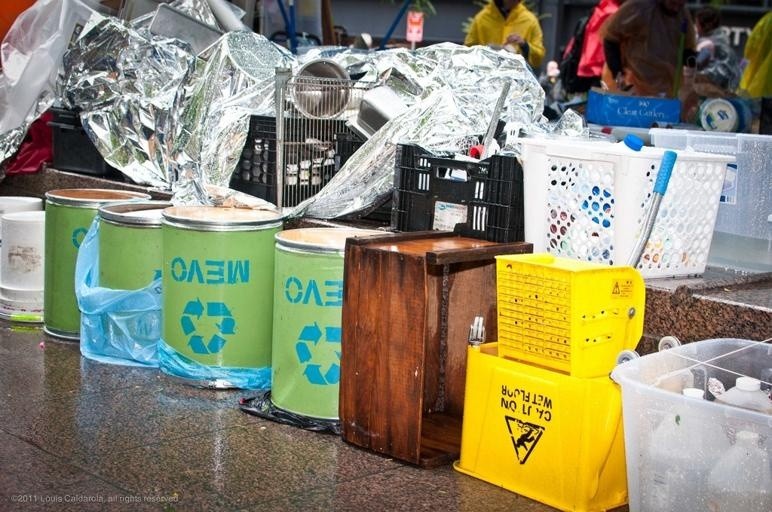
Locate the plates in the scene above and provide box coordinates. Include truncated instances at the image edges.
[0,279,43,324]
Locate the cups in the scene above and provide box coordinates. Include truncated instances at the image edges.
[0,195,45,287]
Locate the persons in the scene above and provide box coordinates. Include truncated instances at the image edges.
[733,12,772,133]
[605,0,696,97]
[462,0,546,67]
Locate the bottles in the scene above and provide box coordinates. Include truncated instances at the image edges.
[567,133,643,257]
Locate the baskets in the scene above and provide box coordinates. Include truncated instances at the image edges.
[390,137,736,279]
[521,138,736,278]
[391,143,524,244]
[229,115,365,207]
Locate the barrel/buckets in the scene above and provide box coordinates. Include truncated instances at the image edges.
[270,225,390,419]
[0,197,46,325]
[41,189,153,343]
[158,207,284,370]
[95,201,170,341]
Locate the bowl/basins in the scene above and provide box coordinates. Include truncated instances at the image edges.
[289,59,352,124]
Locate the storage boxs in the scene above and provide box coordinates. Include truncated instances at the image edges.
[610,335,771,512]
[649,128,771,241]
